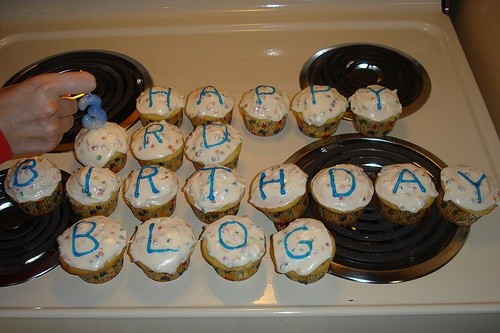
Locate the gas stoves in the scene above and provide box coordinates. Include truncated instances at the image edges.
[0,0,500,332]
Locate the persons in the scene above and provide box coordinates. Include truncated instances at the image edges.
[0,71,96,165]
[454,0,500,140]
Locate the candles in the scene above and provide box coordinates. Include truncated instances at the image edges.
[78,92,108,130]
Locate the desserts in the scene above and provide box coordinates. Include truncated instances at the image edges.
[201,215,267,281]
[63,86,440,226]
[4,156,62,215]
[128,216,198,282]
[56,215,128,284]
[436,165,500,225]
[272,218,337,284]
